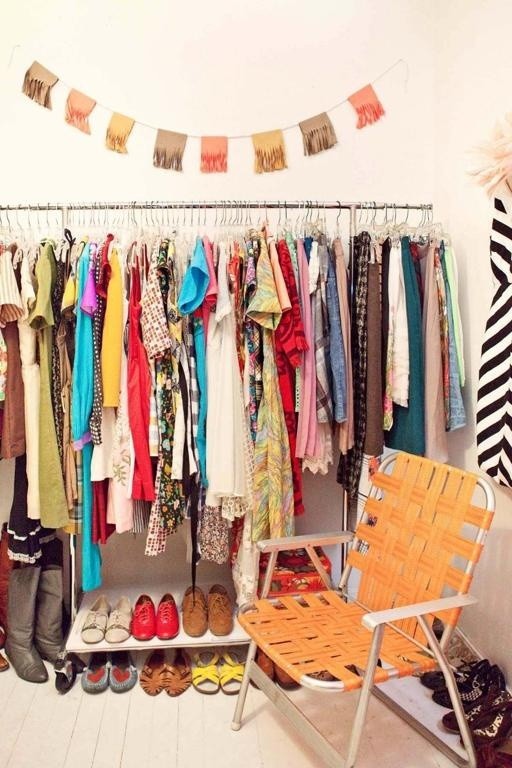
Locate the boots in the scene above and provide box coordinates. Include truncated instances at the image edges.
[34,563,67,667]
[5,565,48,684]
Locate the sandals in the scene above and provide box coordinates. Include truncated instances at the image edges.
[191,646,223,694]
[219,648,247,694]
[421,635,512,768]
[103,595,133,644]
[80,594,110,644]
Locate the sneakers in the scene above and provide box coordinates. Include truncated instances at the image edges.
[107,651,139,693]
[253,646,337,689]
[80,652,109,694]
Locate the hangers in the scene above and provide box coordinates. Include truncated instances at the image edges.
[0,198,452,263]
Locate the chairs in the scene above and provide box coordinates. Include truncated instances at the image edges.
[228,448,499,768]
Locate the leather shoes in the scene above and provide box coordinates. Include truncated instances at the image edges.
[154,592,180,640]
[164,648,194,696]
[140,650,167,697]
[205,584,235,636]
[181,585,208,638]
[133,592,157,642]
